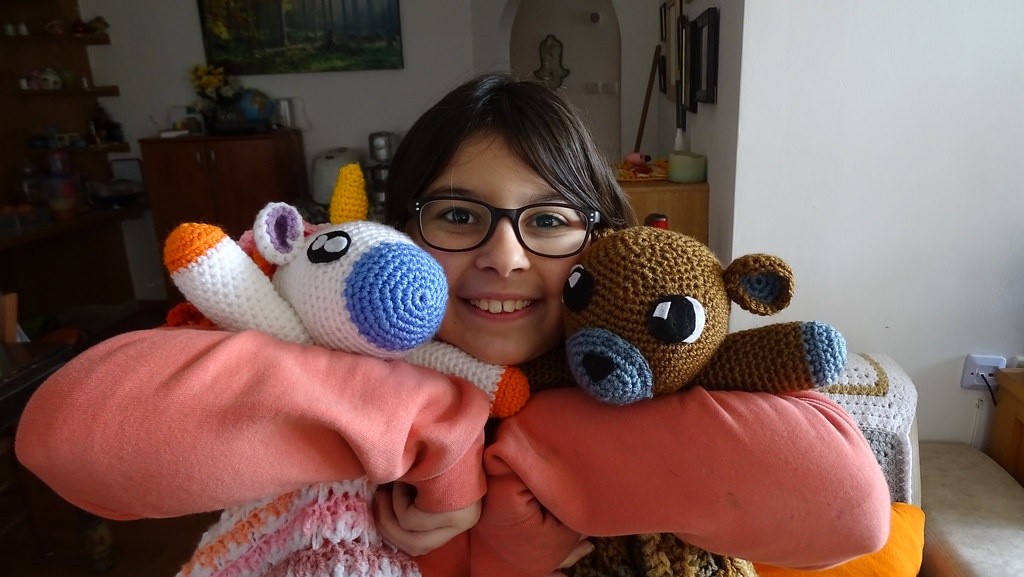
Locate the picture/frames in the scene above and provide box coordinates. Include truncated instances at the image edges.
[691,6,717,104]
[680,22,699,115]
[658,56,666,93]
[659,2,666,41]
[676,14,688,133]
[665,0,682,104]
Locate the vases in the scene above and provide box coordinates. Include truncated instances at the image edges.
[202,97,218,129]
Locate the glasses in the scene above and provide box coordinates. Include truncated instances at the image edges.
[410,195,601,257]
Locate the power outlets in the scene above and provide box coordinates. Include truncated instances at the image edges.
[961,354,1006,391]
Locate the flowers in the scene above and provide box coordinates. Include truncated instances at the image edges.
[183,63,234,99]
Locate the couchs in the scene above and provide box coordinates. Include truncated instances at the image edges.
[816,352,1024,577]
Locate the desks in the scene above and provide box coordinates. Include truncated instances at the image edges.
[0,197,145,316]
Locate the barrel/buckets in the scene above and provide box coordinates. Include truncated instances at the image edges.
[311,148,354,206]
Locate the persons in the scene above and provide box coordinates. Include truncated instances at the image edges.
[15,73,891,577]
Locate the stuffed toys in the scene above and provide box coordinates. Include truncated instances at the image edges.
[550,225,847,577]
[163,163,530,577]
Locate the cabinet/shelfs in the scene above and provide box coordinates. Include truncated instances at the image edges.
[7,33,130,153]
[617,164,710,246]
[139,129,310,300]
